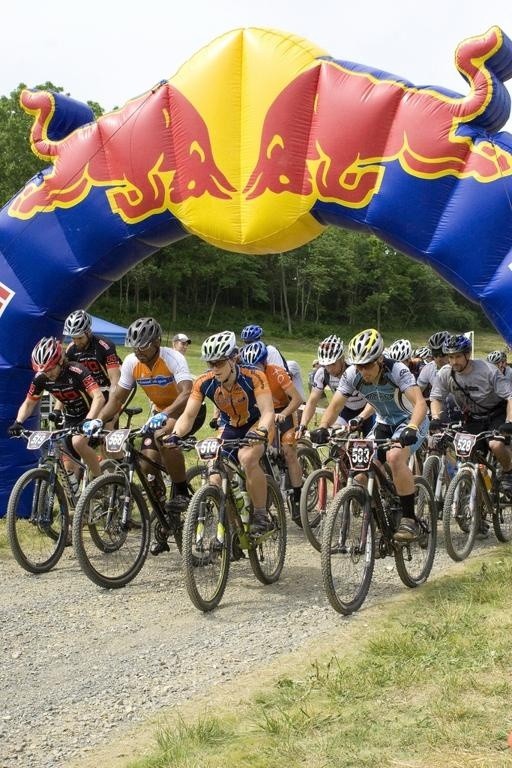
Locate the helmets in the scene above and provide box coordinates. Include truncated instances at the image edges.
[344,328,384,365]
[31,335,63,373]
[428,331,450,350]
[486,351,507,363]
[317,334,344,366]
[124,317,162,348]
[201,331,239,361]
[238,341,268,365]
[442,335,472,354]
[382,339,433,361]
[241,324,263,342]
[62,309,93,338]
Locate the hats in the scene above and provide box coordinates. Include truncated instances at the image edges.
[172,333,192,344]
[311,360,319,365]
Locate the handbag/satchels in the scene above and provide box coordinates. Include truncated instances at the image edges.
[488,401,507,431]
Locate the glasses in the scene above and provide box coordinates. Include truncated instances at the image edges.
[356,361,377,370]
[207,357,230,369]
[433,350,446,358]
[133,342,153,352]
[320,360,336,369]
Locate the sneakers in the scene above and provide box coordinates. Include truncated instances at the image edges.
[475,521,490,539]
[499,474,512,506]
[164,494,191,513]
[393,517,421,542]
[292,501,300,521]
[250,515,276,538]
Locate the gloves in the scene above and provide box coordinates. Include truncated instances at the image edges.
[209,418,220,430]
[48,410,61,423]
[71,419,92,435]
[398,425,418,448]
[8,421,25,436]
[310,427,331,444]
[140,413,168,434]
[82,419,104,438]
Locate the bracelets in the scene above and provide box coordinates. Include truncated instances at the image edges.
[408,425,417,428]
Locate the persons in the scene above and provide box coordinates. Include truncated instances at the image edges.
[310,329,430,559]
[83,319,206,515]
[162,331,275,550]
[8,336,106,542]
[62,309,122,496]
[169,324,510,521]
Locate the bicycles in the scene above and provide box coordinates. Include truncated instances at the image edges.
[287,421,451,556]
[314,433,438,617]
[72,421,224,589]
[164,433,287,612]
[439,423,512,562]
[6,419,135,574]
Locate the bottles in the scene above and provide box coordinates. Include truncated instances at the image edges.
[146,473,167,503]
[228,479,247,516]
[477,462,493,493]
